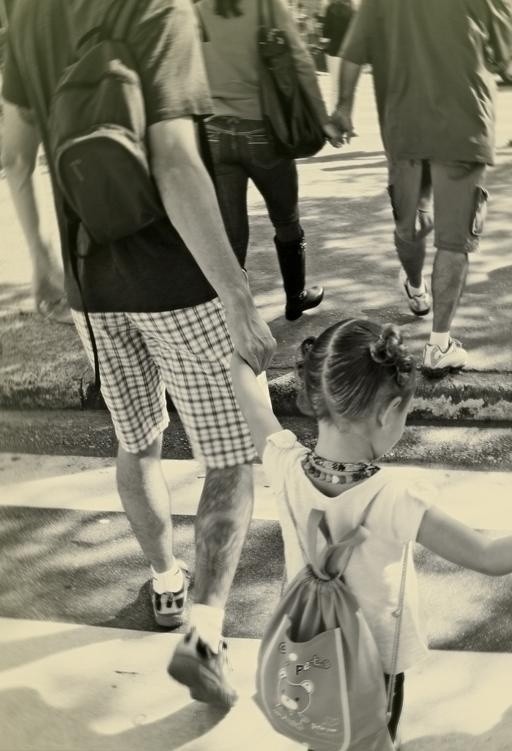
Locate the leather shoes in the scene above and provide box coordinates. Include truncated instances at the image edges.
[285,282,324,321]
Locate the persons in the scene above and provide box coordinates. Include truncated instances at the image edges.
[231,316,512,750]
[0,0,278,710]
[194,0,348,322]
[329,0,512,376]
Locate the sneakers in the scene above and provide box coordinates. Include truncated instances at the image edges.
[398,265,432,316]
[147,557,191,627]
[420,334,468,379]
[168,624,240,709]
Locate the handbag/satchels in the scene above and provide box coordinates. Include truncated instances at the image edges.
[256,20,326,159]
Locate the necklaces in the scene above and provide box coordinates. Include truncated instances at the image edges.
[310,448,372,471]
[301,452,379,484]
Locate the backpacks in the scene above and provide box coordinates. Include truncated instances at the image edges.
[47,0,209,404]
[251,461,418,750]
